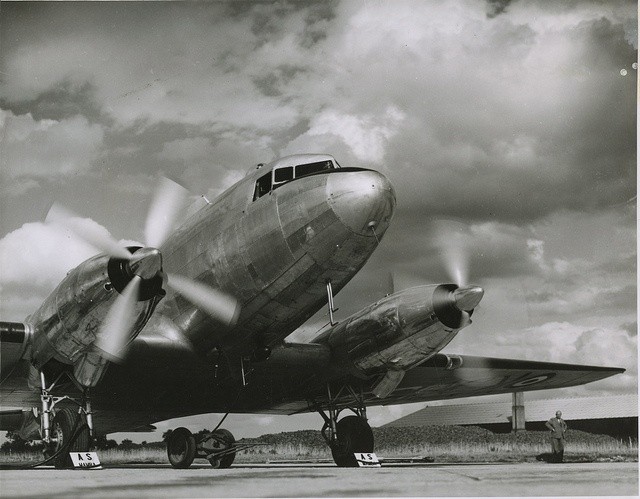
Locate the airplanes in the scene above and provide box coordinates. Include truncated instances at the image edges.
[0,155,625,470]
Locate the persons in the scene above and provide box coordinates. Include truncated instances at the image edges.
[545,410,567,463]
